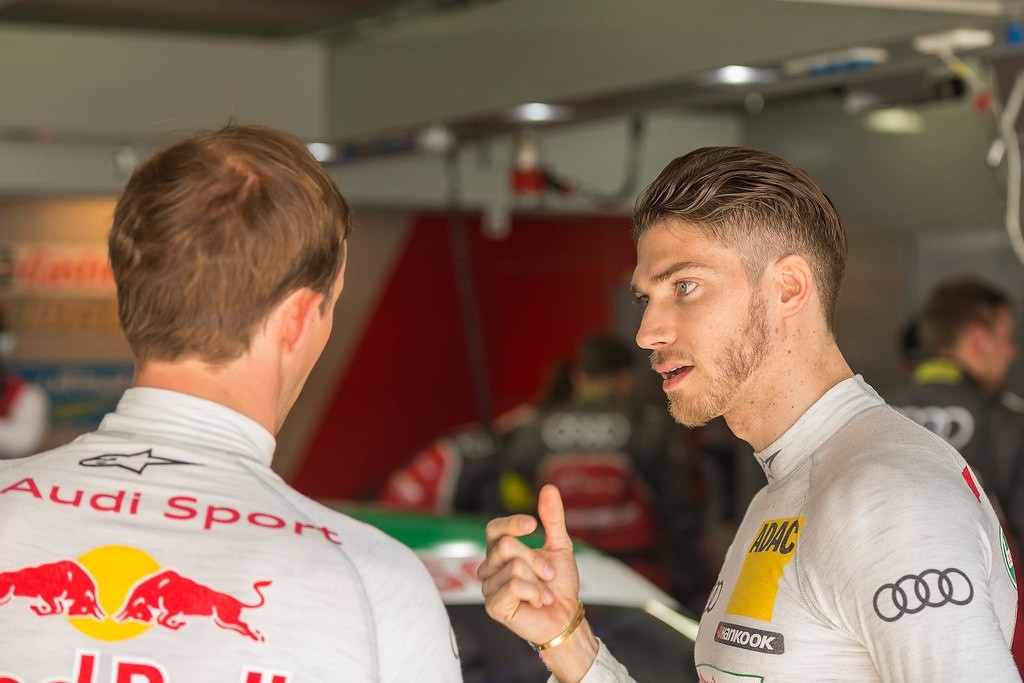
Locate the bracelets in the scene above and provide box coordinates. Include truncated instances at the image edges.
[525,604,585,652]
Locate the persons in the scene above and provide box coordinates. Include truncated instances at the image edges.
[876,279,1024,552]
[496,335,699,614]
[0,313,53,460]
[475,144,1021,683]
[0,117,469,683]
[897,315,1023,416]
[546,354,713,607]
[379,401,543,517]
